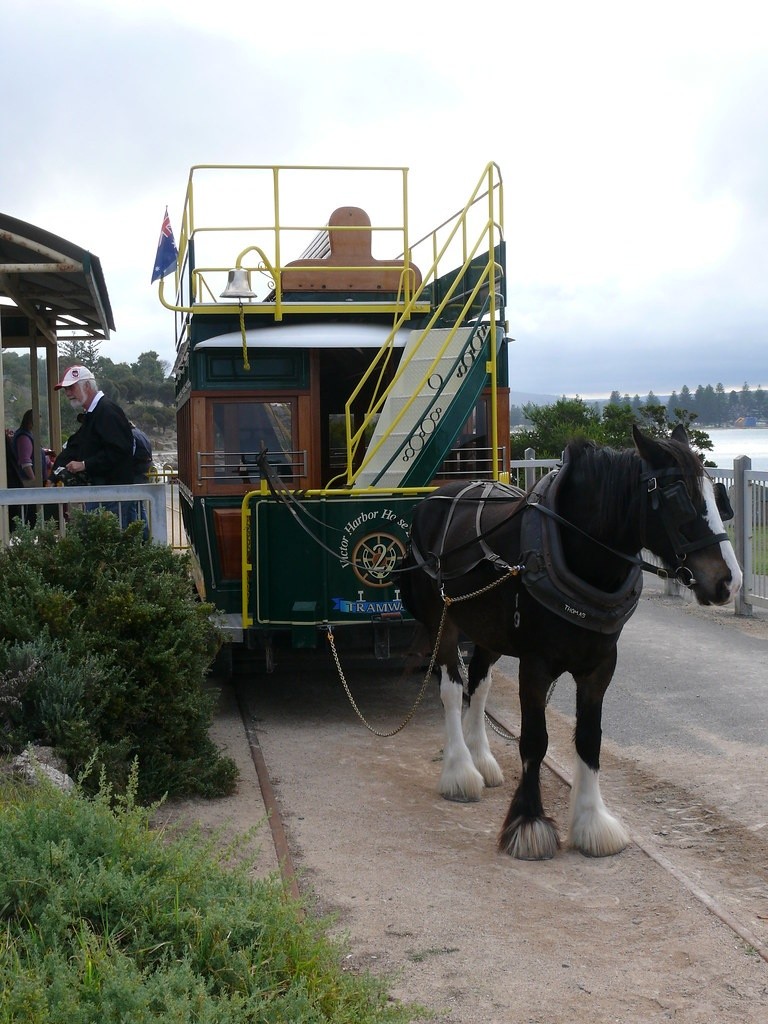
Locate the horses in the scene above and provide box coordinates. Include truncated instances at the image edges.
[397,420,745,861]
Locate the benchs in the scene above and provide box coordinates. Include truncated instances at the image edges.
[262,206,422,302]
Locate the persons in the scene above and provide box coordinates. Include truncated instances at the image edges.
[10,409,59,541]
[127,421,152,545]
[43,365,131,571]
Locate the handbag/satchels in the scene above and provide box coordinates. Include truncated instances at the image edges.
[133,436,152,463]
[43,469,91,486]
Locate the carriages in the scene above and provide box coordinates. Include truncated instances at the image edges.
[159,161,744,861]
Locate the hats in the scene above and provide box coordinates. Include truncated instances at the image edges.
[53,366,95,391]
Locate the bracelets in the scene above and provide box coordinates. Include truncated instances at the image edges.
[83,460,85,465]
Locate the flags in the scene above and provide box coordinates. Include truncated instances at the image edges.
[151,211,179,284]
